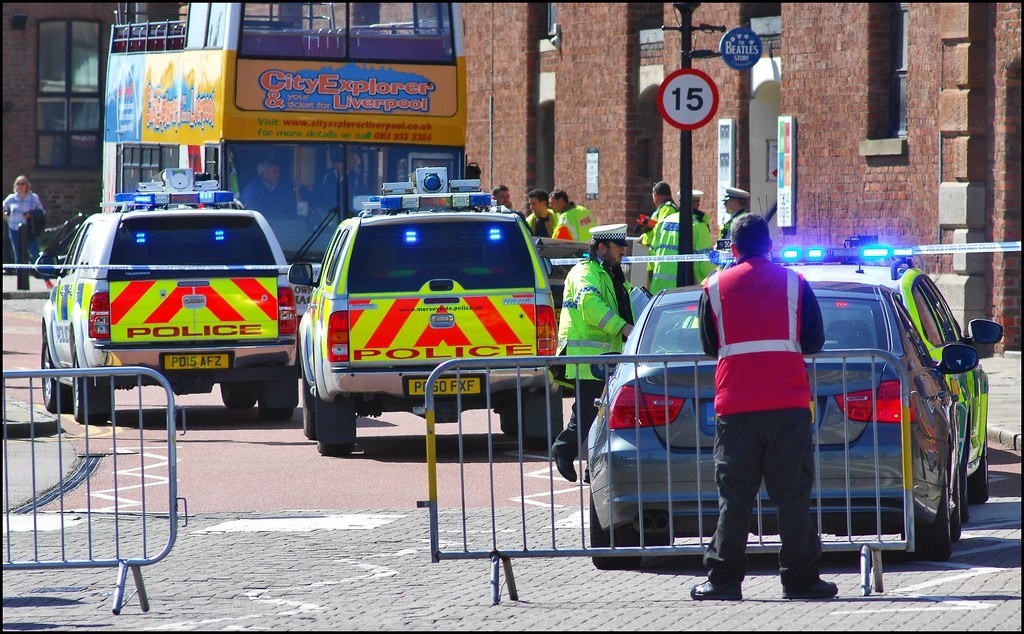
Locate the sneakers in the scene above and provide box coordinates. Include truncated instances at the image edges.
[551,444,578,482]
[690,579,742,600]
[585,468,590,484]
[782,573,837,599]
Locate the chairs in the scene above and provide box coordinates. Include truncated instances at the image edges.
[822,320,875,350]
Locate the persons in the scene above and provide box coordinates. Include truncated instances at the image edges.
[238,149,365,216]
[492,181,751,298]
[2,175,54,288]
[689,212,839,600]
[551,223,635,484]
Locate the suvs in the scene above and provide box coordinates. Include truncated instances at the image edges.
[32,168,300,428]
[287,165,569,455]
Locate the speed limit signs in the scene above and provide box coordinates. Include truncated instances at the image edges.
[657,68,719,131]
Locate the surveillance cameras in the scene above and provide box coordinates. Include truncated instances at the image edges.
[551,36,561,48]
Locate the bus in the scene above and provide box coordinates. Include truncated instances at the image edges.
[92,1,467,326]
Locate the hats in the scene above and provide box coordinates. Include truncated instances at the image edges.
[677,189,704,202]
[722,188,750,201]
[653,182,671,197]
[589,224,629,246]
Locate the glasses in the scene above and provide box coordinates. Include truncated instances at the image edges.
[15,182,26,186]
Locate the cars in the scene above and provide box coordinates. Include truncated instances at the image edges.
[644,233,1004,523]
[587,280,978,570]
